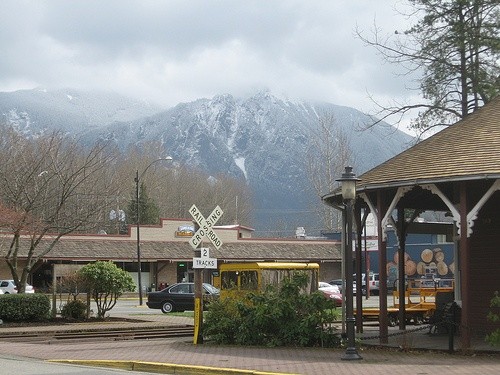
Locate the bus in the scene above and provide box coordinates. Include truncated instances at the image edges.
[217,258,321,305]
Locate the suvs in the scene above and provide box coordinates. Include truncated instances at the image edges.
[353,273,380,296]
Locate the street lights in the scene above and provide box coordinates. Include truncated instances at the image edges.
[334,165,363,361]
[133,156,173,306]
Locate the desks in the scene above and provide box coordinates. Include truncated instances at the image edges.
[355,307,429,326]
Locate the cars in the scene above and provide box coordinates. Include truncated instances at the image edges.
[318,281,341,294]
[328,279,357,296]
[146,282,220,313]
[323,291,347,304]
[0,280,35,295]
